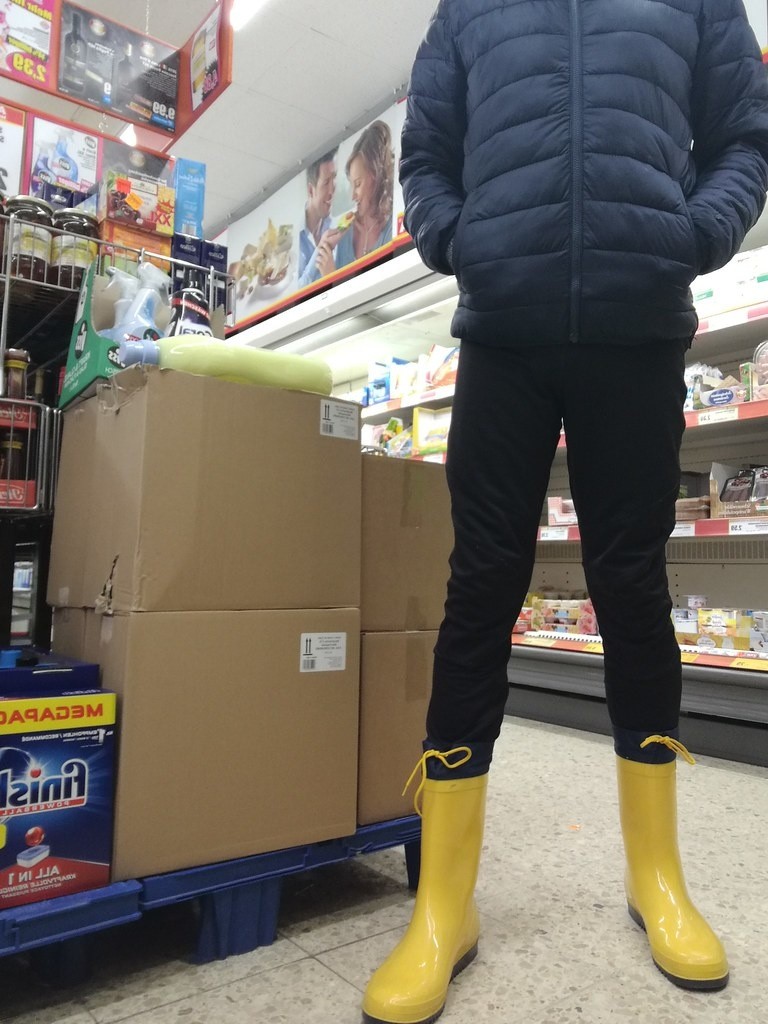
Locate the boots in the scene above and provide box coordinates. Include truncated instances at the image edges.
[360,741,489,1024]
[611,735,730,989]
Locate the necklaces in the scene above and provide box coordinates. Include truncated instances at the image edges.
[363,216,378,255]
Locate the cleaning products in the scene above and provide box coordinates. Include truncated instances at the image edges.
[96,262,332,394]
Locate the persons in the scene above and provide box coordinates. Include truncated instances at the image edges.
[298,120,395,288]
[362,0,768,1024]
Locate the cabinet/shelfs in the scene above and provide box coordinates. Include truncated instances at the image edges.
[362,295,768,673]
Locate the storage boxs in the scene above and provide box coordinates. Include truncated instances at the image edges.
[362,451,454,632]
[0,687,117,911]
[56,253,169,411]
[49,611,359,882]
[47,363,362,613]
[359,631,442,828]
